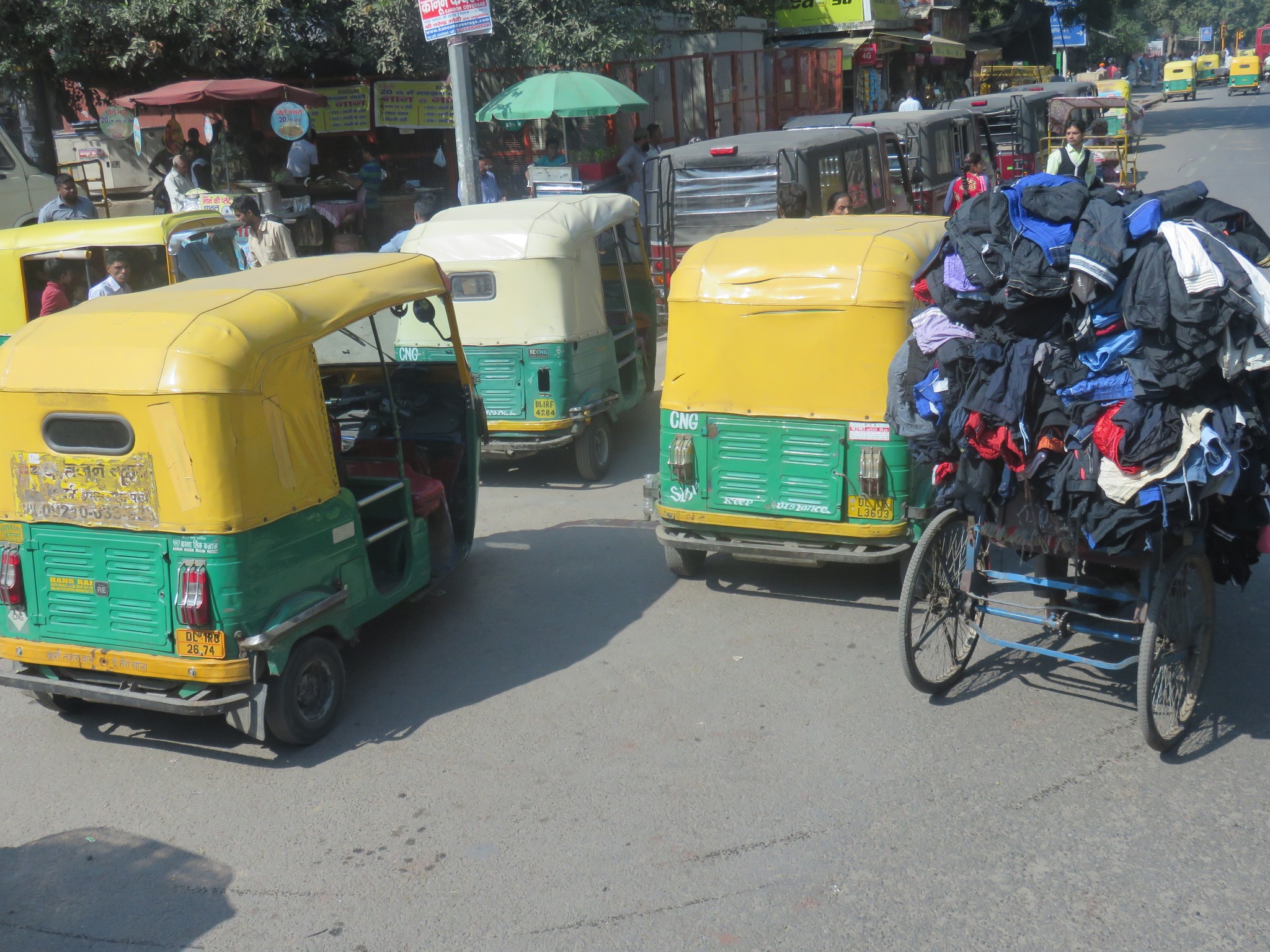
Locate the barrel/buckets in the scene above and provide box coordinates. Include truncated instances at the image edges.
[258,190,283,214]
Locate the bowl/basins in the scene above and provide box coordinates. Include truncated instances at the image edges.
[251,183,280,193]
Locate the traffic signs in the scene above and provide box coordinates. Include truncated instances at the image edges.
[1044,0,1088,48]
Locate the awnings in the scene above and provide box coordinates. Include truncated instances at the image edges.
[774,36,868,58]
[128,76,331,194]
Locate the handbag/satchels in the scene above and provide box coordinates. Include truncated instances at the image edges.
[433,148,447,168]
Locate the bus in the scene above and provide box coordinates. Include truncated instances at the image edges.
[1254,23,1270,74]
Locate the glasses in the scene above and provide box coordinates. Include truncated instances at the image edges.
[479,163,493,169]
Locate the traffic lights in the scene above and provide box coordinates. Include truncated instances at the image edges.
[1221,25,1227,39]
[1238,27,1244,40]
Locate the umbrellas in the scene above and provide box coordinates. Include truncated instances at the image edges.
[475,69,650,171]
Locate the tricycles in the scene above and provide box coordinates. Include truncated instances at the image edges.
[389,189,660,484]
[887,414,1220,751]
[649,208,964,600]
[1084,79,1136,152]
[1195,53,1220,86]
[0,254,490,755]
[1236,46,1255,57]
[1227,55,1261,96]
[1161,59,1197,102]
[1,208,251,360]
[1035,94,1146,193]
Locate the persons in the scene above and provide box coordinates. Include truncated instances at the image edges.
[778,182,808,219]
[1032,551,1143,634]
[827,191,855,216]
[1045,118,1097,191]
[86,249,138,303]
[457,148,508,208]
[38,257,74,318]
[620,124,668,264]
[941,151,992,217]
[1083,119,1125,184]
[377,192,441,253]
[230,194,299,270]
[142,244,169,291]
[526,139,574,200]
[147,117,330,215]
[334,141,387,252]
[875,0,1270,115]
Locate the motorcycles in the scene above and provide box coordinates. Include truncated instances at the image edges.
[1263,62,1270,83]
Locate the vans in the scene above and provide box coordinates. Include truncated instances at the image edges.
[0,127,91,247]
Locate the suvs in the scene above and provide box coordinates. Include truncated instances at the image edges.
[637,122,917,331]
[838,108,998,222]
[937,88,1064,187]
[1007,82,1113,136]
